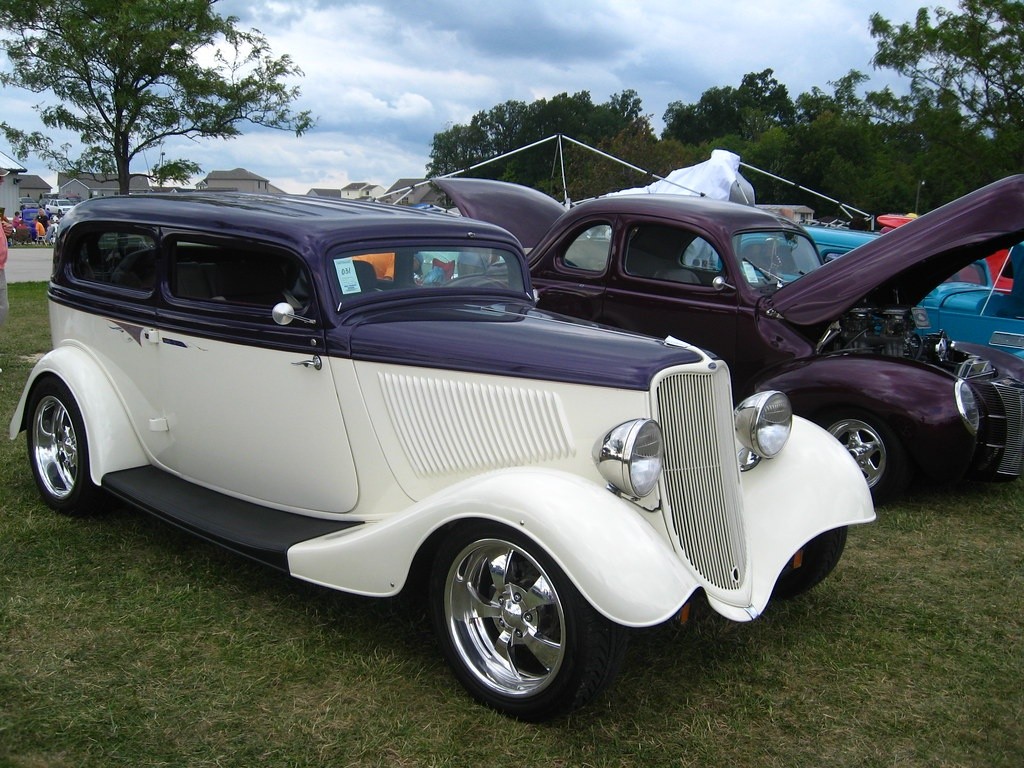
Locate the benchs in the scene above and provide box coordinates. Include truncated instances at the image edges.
[112,246,234,304]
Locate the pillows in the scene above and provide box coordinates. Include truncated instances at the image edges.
[201,263,229,297]
[145,262,210,298]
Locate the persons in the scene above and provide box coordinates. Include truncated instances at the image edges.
[0,217,9,322]
[352,250,491,286]
[0,204,51,244]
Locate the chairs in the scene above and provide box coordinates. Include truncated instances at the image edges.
[353,262,377,292]
[662,267,702,283]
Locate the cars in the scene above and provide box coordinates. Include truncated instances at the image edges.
[21,209,49,240]
[20,196,40,211]
[2,192,880,729]
[426,168,1024,509]
[45,198,75,216]
